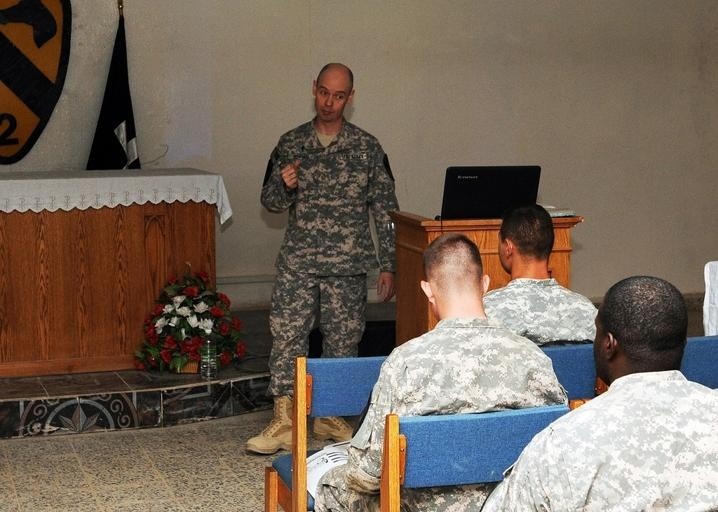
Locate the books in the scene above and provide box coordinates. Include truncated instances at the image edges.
[307,440,350,499]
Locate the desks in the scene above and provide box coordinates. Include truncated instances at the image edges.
[0,167,221,377]
[388,211,583,350]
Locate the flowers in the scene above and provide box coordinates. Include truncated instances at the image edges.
[134,271,247,372]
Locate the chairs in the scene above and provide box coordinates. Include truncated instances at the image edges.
[376,399,583,512]
[538,345,596,398]
[265,357,386,511]
[680,334,715,389]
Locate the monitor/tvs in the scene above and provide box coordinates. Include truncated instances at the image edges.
[441,165,541,221]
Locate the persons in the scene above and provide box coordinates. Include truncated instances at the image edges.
[481,275,718,512]
[313,234,569,512]
[482,203,601,345]
[246,62,397,456]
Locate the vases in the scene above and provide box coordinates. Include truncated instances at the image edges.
[181,361,200,373]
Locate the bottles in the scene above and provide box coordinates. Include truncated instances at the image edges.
[201,340,218,381]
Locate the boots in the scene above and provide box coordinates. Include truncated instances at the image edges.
[247,394,308,454]
[313,416,355,442]
